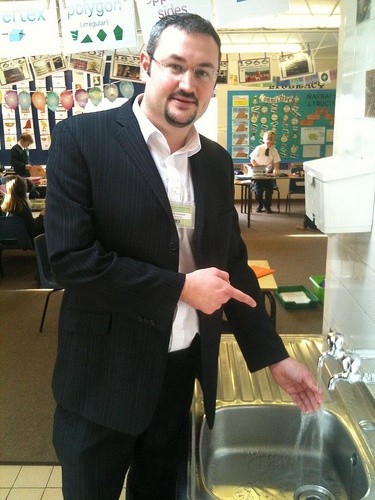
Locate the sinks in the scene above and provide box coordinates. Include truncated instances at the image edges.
[200,403,370,500]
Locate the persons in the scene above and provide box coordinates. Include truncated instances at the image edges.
[249,130,282,214]
[0,162,45,249]
[10,132,40,198]
[123,66,131,78]
[220,73,223,78]
[74,59,83,69]
[10,72,18,82]
[44,14,324,500]
[247,72,264,81]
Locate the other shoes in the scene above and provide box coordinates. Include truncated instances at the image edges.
[265,207,271,213]
[256,205,263,212]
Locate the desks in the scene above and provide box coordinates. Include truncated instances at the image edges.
[248,259,278,330]
[235,174,305,227]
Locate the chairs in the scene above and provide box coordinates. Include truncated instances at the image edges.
[0,215,34,276]
[251,179,280,212]
[33,233,65,333]
[285,167,304,215]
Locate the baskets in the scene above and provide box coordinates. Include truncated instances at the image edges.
[275,284,320,310]
[309,276,325,303]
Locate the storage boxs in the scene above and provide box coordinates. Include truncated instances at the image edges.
[274,285,320,310]
[310,276,325,303]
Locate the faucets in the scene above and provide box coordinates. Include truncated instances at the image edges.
[327,353,365,389]
[317,331,349,371]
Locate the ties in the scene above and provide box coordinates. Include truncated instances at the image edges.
[265,149,269,157]
